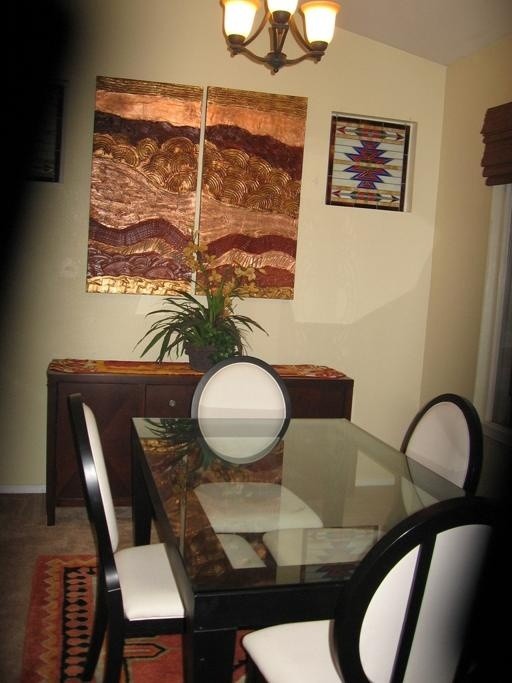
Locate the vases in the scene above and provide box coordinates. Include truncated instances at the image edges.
[185,344,221,372]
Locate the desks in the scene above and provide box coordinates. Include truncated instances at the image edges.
[126,417,461,680]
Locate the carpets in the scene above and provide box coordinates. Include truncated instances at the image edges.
[18,555,252,683]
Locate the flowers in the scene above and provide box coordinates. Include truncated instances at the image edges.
[133,228,268,365]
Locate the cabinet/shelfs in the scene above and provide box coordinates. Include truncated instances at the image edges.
[46,358,350,526]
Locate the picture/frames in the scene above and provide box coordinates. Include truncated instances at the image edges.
[325,111,417,213]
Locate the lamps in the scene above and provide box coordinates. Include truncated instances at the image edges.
[220,0,343,73]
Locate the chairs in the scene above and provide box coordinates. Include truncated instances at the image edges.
[241,494,494,679]
[187,364,330,531]
[70,400,265,683]
[260,393,485,589]
